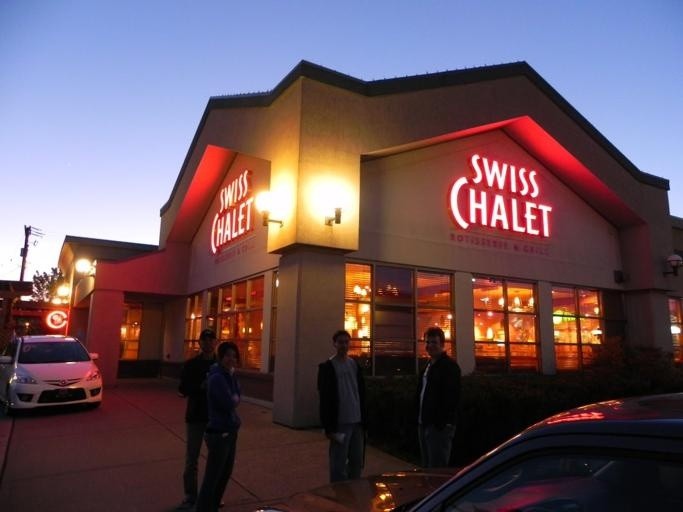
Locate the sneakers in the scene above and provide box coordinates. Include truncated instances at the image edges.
[219,497,224,507]
[176,496,196,509]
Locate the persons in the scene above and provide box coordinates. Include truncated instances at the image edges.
[175,326,221,511]
[414,326,463,469]
[195,339,243,511]
[315,329,367,484]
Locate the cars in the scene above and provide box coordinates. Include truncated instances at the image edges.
[0,332,103,410]
[389,389,682,510]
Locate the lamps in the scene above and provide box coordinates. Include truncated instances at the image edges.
[50,285,71,305]
[254,191,285,228]
[75,258,96,277]
[663,255,683,277]
[319,184,349,228]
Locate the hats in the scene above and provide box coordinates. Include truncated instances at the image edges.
[200,329,216,339]
